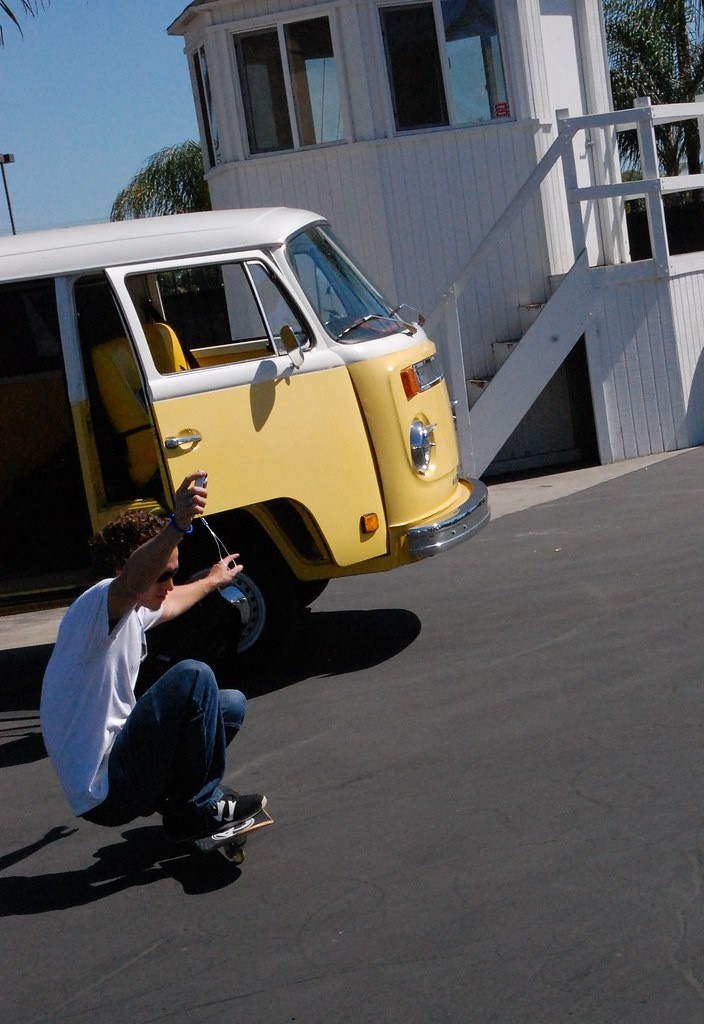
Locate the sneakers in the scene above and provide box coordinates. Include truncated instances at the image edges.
[162,794,268,843]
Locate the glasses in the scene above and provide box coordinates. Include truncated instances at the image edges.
[156,567,178,583]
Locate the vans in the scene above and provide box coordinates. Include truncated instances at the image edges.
[0,199,495,668]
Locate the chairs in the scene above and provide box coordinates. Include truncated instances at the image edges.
[93,323,194,493]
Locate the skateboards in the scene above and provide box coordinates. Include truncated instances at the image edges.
[165,783,277,867]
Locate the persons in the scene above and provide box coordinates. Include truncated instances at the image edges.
[38,471,268,843]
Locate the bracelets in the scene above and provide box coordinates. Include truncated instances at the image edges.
[167,513,194,535]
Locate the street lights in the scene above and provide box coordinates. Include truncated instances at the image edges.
[0,152,17,235]
